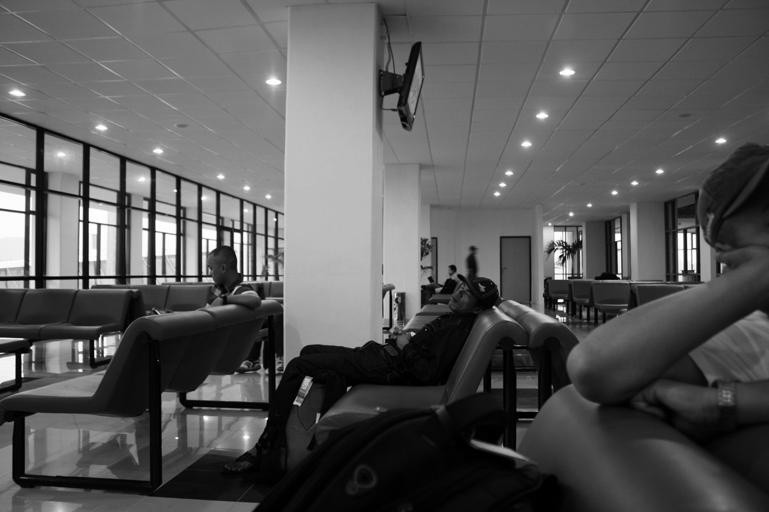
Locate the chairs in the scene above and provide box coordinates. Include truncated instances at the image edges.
[311,271,732,506]
[2,271,285,494]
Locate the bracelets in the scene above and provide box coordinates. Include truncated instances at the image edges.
[711,378,742,434]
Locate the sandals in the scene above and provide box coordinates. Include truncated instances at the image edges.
[223,443,261,475]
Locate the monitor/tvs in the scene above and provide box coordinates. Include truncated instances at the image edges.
[397,42,425,131]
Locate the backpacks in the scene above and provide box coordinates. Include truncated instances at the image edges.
[254,389,572,512]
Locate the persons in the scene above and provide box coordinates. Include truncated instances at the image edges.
[434,245,479,295]
[146,246,284,375]
[223,274,499,474]
[567,143,769,443]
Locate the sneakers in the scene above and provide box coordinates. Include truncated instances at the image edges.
[235,359,261,373]
[264,359,284,375]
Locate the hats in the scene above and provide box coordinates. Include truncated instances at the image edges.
[696,143,768,248]
[457,273,498,308]
